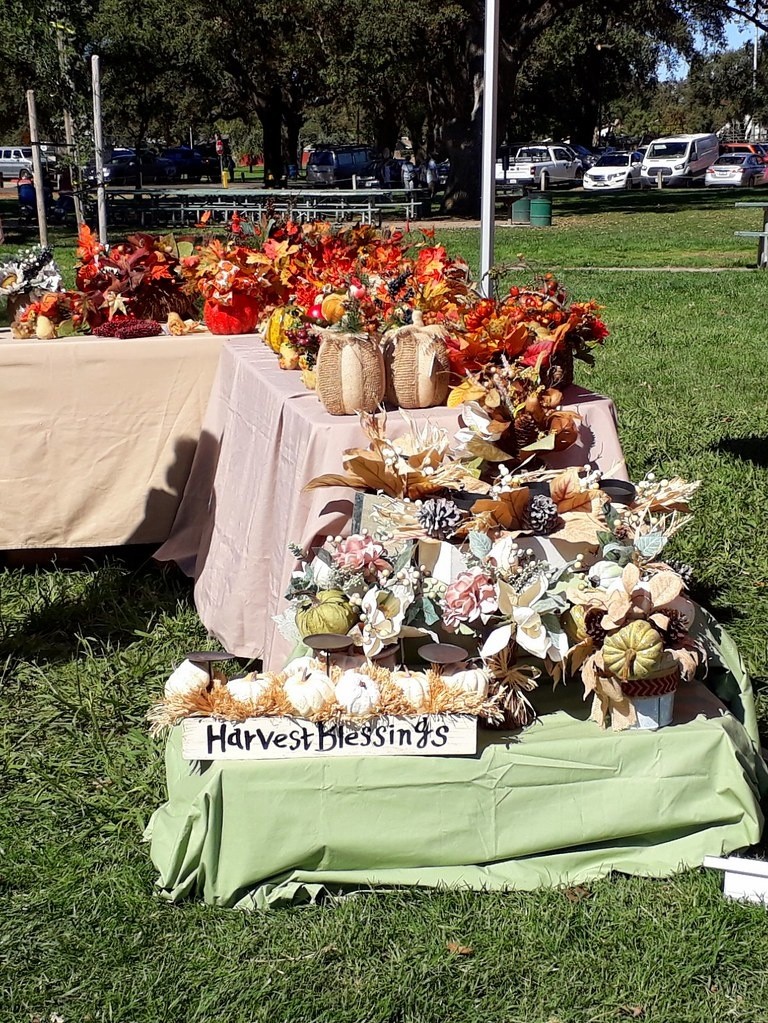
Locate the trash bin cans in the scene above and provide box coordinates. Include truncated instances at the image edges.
[529,190,552,228]
[512,188,530,225]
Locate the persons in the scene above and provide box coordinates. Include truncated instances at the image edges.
[401,155,415,190]
[426,152,440,200]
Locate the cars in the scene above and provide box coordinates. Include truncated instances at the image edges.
[495,137,649,171]
[582,151,644,193]
[706,152,768,188]
[81,154,176,187]
[101,135,454,195]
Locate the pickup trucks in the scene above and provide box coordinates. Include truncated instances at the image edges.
[494,145,583,190]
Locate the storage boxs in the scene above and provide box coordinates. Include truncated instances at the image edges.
[511,196,554,226]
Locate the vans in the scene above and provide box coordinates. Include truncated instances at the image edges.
[640,133,717,191]
[0,147,51,180]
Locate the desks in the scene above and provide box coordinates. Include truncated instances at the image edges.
[732,199,767,267]
[0,317,633,673]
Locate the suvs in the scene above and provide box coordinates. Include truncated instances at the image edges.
[720,142,767,163]
